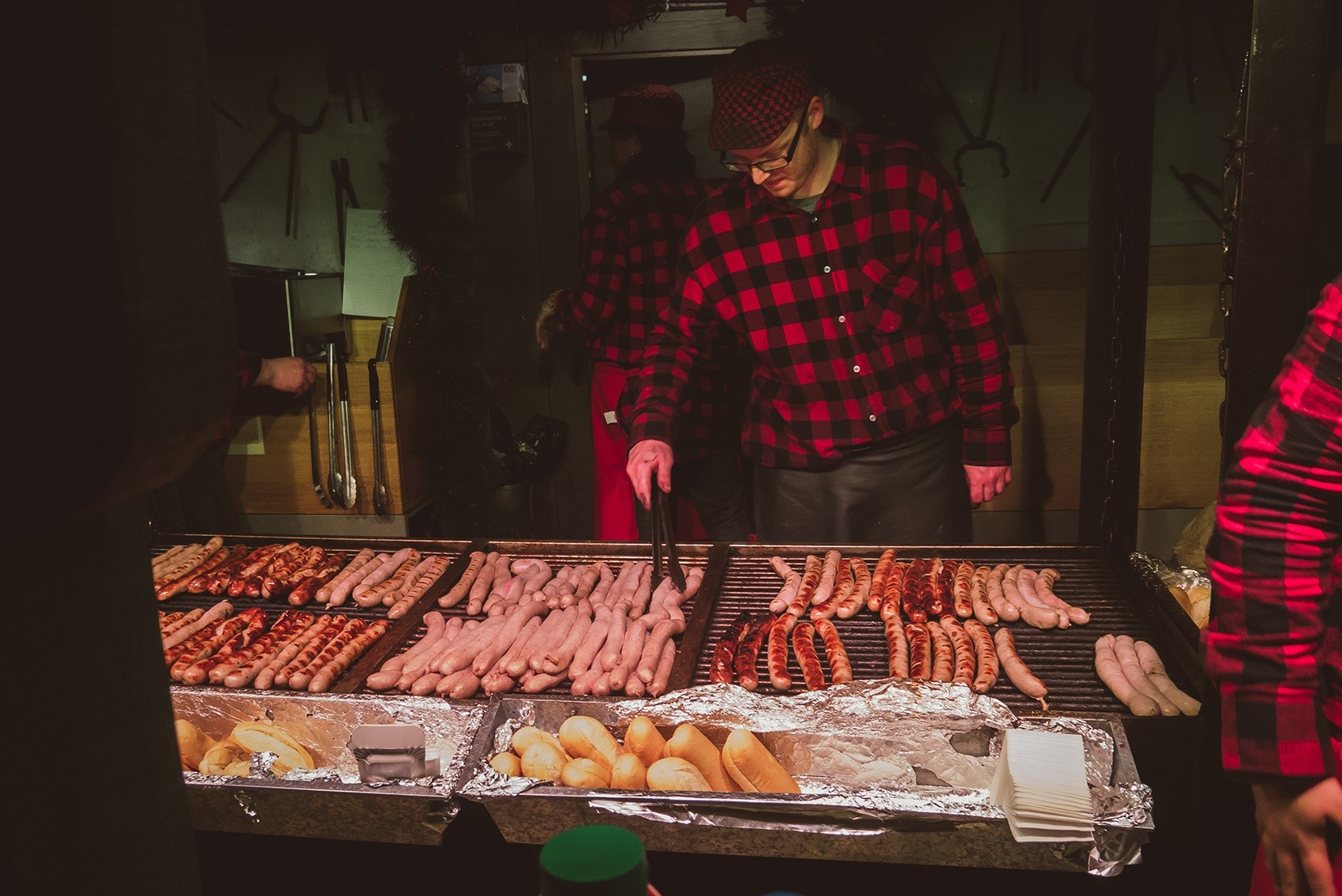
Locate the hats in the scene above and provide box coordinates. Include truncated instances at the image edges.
[597,83,685,131]
[708,38,818,150]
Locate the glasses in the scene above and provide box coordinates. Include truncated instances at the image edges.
[719,100,808,173]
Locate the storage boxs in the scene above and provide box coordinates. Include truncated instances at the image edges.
[454,694,1157,879]
[169,682,493,846]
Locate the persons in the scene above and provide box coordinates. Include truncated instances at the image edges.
[1204,269,1342,896]
[623,40,1023,546]
[179,345,319,534]
[533,83,730,542]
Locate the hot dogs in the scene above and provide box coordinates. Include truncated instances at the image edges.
[483,712,801,796]
[173,719,315,777]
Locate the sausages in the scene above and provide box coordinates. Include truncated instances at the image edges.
[148,535,1206,716]
